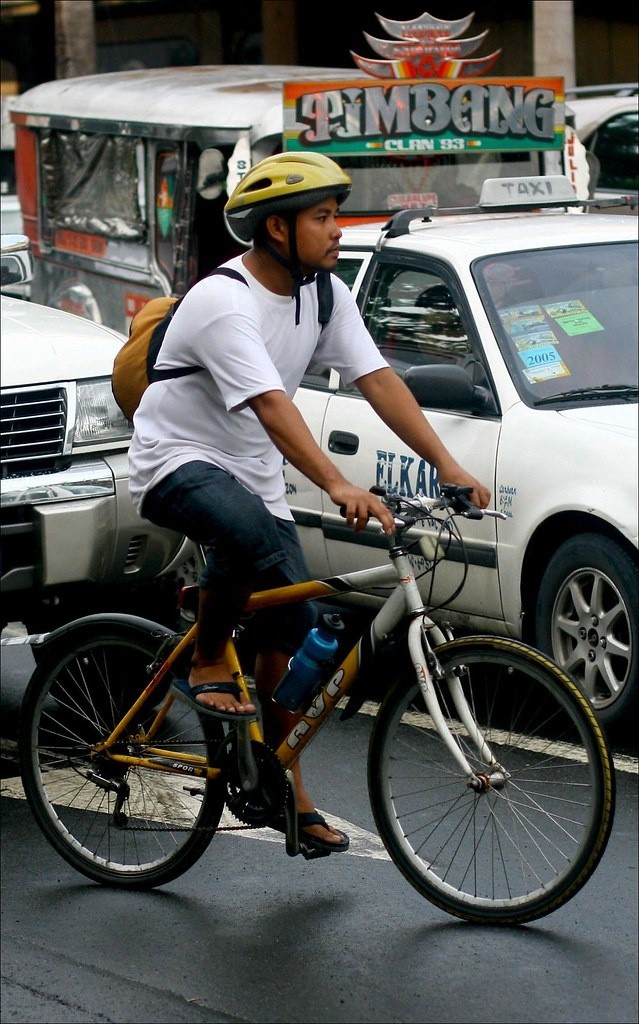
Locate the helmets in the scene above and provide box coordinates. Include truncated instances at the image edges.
[415,285,457,319]
[223,150,349,246]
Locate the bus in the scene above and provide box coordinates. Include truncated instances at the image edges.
[8,61,600,344]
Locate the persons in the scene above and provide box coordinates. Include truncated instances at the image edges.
[127,151,490,853]
[397,286,486,386]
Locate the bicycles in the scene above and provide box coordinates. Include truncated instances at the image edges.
[17,479,617,928]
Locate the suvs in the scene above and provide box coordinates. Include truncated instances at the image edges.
[461,82,638,216]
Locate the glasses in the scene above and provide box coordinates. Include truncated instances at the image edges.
[429,319,463,334]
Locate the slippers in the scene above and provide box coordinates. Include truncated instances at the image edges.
[169,682,259,719]
[267,810,350,851]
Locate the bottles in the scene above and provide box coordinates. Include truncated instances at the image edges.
[272,613,345,712]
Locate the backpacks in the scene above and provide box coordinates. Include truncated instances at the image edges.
[110,266,336,424]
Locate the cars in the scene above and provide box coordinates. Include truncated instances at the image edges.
[0,84,639,745]
